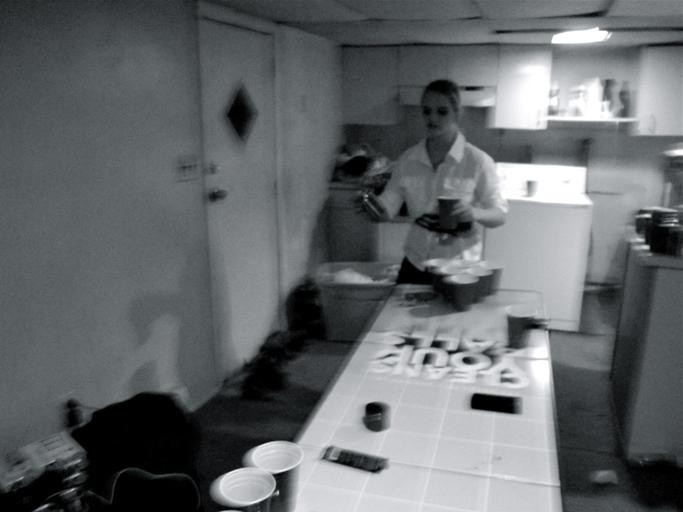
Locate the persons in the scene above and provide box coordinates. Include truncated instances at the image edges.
[351,78,510,285]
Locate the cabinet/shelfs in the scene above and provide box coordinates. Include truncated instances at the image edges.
[485,44,551,131]
[342,44,400,126]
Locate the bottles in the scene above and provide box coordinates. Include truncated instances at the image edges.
[355,188,387,222]
[550,71,616,120]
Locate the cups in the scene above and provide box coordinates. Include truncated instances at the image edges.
[526,180,538,197]
[437,196,464,232]
[208,438,303,511]
[633,202,682,257]
[424,252,504,312]
[503,303,539,351]
